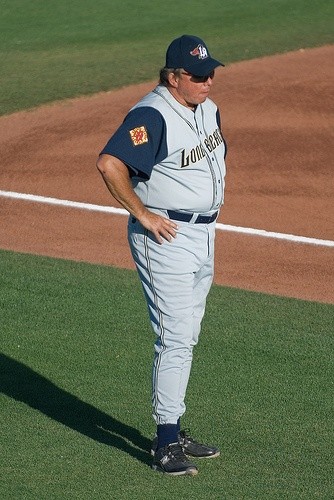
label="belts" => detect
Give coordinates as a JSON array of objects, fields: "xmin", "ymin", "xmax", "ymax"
[{"xmin": 167, "ymin": 208, "xmax": 218, "ymax": 224}]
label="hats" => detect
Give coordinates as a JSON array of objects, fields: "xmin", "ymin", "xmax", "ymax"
[{"xmin": 165, "ymin": 34, "xmax": 226, "ymax": 77}]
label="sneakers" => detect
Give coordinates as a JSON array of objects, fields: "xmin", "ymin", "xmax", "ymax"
[
  {"xmin": 151, "ymin": 437, "xmax": 198, "ymax": 476},
  {"xmin": 177, "ymin": 428, "xmax": 221, "ymax": 459}
]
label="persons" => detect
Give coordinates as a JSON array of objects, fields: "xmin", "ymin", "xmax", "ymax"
[{"xmin": 97, "ymin": 35, "xmax": 228, "ymax": 477}]
